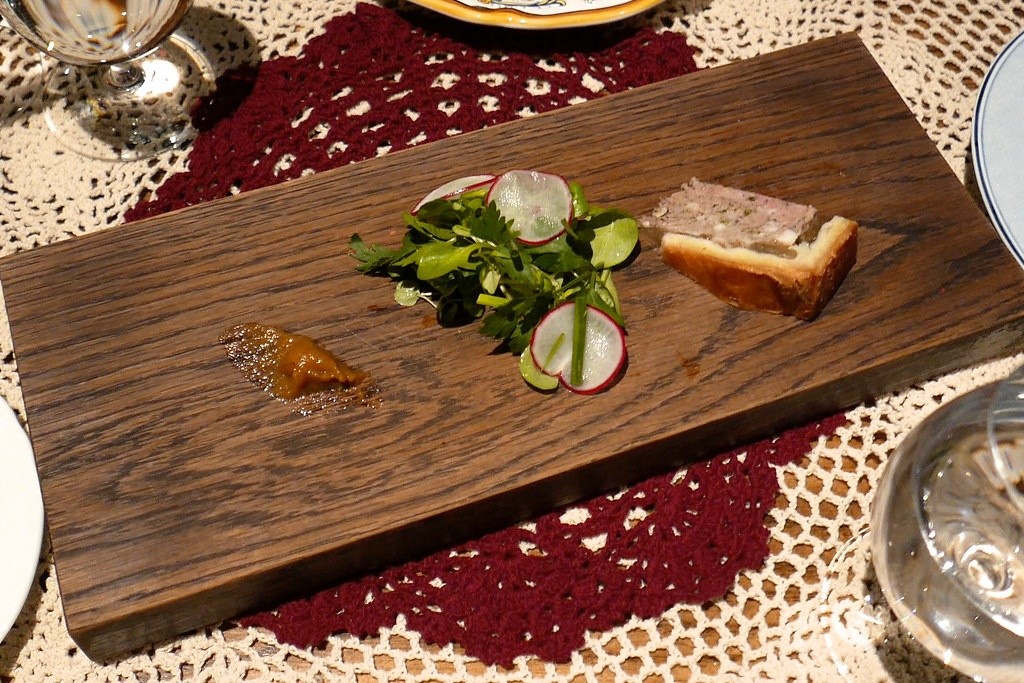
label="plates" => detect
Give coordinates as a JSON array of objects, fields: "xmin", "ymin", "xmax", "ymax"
[
  {"xmin": 405, "ymin": 0, "xmax": 666, "ymax": 30},
  {"xmin": 0, "ymin": 398, "xmax": 45, "ymax": 647},
  {"xmin": 973, "ymin": 31, "xmax": 1023, "ymax": 269}
]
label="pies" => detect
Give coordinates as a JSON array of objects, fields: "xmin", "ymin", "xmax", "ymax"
[{"xmin": 640, "ymin": 177, "xmax": 859, "ymax": 321}]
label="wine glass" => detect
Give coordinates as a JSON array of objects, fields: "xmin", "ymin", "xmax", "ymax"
[
  {"xmin": 819, "ymin": 366, "xmax": 1023, "ymax": 683},
  {"xmin": 0, "ymin": 0, "xmax": 215, "ymax": 162}
]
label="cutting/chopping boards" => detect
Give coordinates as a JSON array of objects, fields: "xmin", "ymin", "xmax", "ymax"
[{"xmin": 2, "ymin": 32, "xmax": 1024, "ymax": 668}]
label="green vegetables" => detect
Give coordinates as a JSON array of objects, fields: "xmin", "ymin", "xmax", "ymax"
[{"xmin": 347, "ymin": 179, "xmax": 639, "ymax": 391}]
